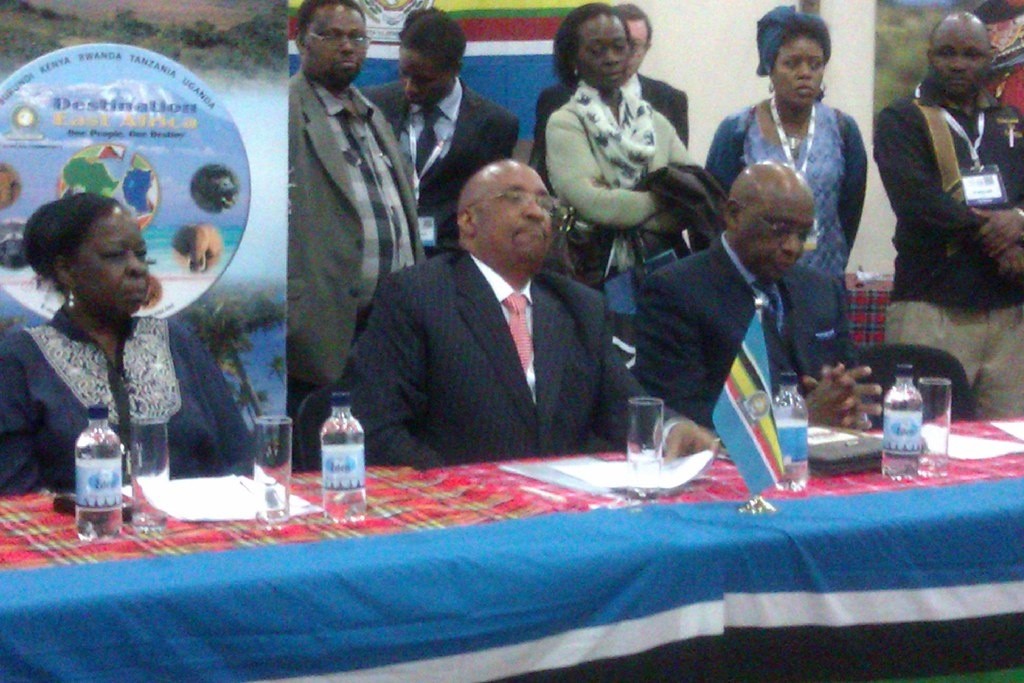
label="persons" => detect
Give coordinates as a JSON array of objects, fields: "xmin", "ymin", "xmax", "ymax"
[
  {"xmin": 344, "ymin": 159, "xmax": 722, "ymax": 466},
  {"xmin": 637, "ymin": 160, "xmax": 895, "ymax": 431},
  {"xmin": 362, "ymin": 8, "xmax": 520, "ymax": 258},
  {"xmin": 530, "ymin": 5, "xmax": 690, "ymax": 284},
  {"xmin": 875, "ymin": 12, "xmax": 1024, "ymax": 419},
  {"xmin": 0, "ymin": 193, "xmax": 254, "ymax": 497},
  {"xmin": 704, "ymin": 8, "xmax": 868, "ymax": 382},
  {"xmin": 288, "ymin": 1, "xmax": 422, "ymax": 419}
]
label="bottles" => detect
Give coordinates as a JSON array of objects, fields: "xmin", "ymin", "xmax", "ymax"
[
  {"xmin": 75, "ymin": 403, "xmax": 120, "ymax": 542},
  {"xmin": 882, "ymin": 364, "xmax": 925, "ymax": 485},
  {"xmin": 320, "ymin": 391, "xmax": 366, "ymax": 524},
  {"xmin": 772, "ymin": 372, "xmax": 809, "ymax": 490}
]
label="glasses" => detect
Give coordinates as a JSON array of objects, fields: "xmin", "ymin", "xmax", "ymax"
[
  {"xmin": 464, "ymin": 189, "xmax": 556, "ymax": 215},
  {"xmin": 307, "ymin": 32, "xmax": 371, "ymax": 49},
  {"xmin": 741, "ymin": 202, "xmax": 821, "ymax": 243}
]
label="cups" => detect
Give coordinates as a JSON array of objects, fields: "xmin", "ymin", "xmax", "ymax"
[
  {"xmin": 918, "ymin": 376, "xmax": 952, "ymax": 477},
  {"xmin": 626, "ymin": 398, "xmax": 664, "ymax": 484},
  {"xmin": 130, "ymin": 415, "xmax": 170, "ymax": 537},
  {"xmin": 255, "ymin": 416, "xmax": 293, "ymax": 526}
]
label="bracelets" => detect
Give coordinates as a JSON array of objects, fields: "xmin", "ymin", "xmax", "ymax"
[{"xmin": 1015, "ymin": 207, "xmax": 1024, "ymax": 216}]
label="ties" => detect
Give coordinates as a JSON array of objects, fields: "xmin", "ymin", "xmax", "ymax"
[
  {"xmin": 416, "ymin": 104, "xmax": 444, "ymax": 176},
  {"xmin": 503, "ymin": 293, "xmax": 533, "ymax": 379},
  {"xmin": 754, "ymin": 282, "xmax": 798, "ymax": 398}
]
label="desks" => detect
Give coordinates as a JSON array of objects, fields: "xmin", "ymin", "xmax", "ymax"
[
  {"xmin": 0, "ymin": 416, "xmax": 1023, "ymax": 683},
  {"xmin": 833, "ymin": 271, "xmax": 907, "ymax": 350}
]
